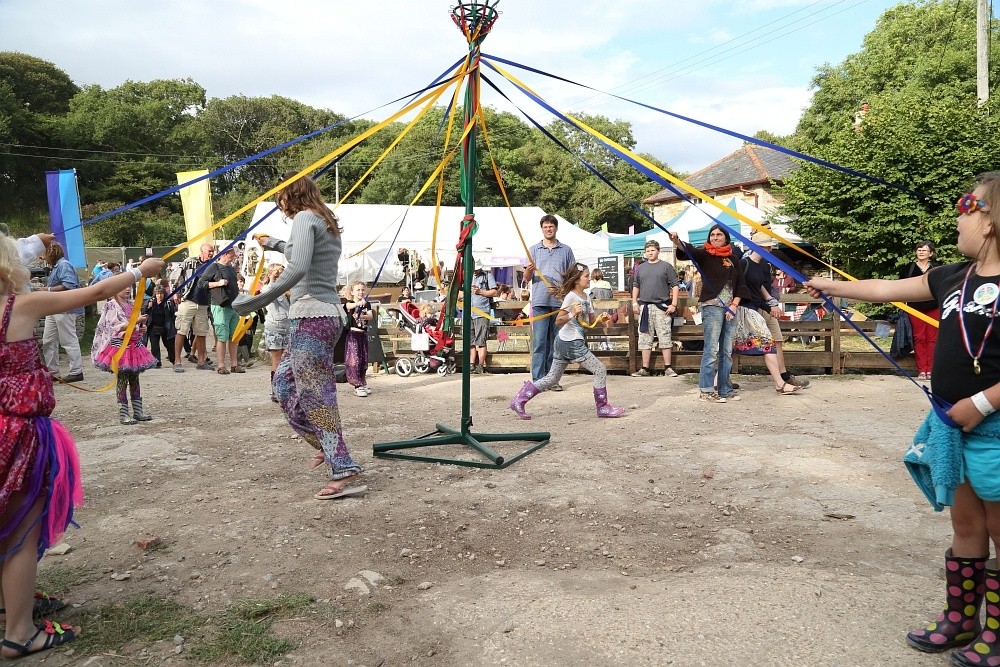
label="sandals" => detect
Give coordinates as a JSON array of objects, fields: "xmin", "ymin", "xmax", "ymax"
[
  {"xmin": 776, "ymin": 382, "xmax": 803, "ymax": 394},
  {"xmin": 0, "ymin": 619, "xmax": 82, "ymax": 661},
  {"xmin": 918, "ymin": 371, "xmax": 931, "ymax": 380},
  {"xmin": 0, "ymin": 588, "xmax": 71, "ymax": 631}
]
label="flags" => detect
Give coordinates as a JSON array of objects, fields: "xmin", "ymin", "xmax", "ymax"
[
  {"xmin": 45, "ymin": 169, "xmax": 87, "ymax": 269},
  {"xmin": 176, "ymin": 170, "xmax": 213, "ymax": 258}
]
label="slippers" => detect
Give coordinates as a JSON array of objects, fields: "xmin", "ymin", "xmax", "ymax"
[
  {"xmin": 307, "ymin": 449, "xmax": 328, "ymax": 472},
  {"xmin": 316, "ymin": 485, "xmax": 367, "ymax": 499}
]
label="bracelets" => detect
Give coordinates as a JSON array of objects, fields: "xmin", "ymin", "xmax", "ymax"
[
  {"xmin": 731, "ymin": 303, "xmax": 738, "ymax": 307},
  {"xmin": 671, "ymin": 304, "xmax": 677, "ymax": 307},
  {"xmin": 971, "ymin": 391, "xmax": 996, "ymax": 417},
  {"xmin": 48, "ymin": 287, "xmax": 51, "ymax": 292},
  {"xmin": 633, "ymin": 302, "xmax": 638, "ymax": 306}
]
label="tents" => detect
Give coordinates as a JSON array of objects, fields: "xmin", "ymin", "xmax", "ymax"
[
  {"xmin": 609, "ymin": 195, "xmax": 811, "ymax": 255},
  {"xmin": 242, "ymin": 199, "xmax": 613, "ymax": 294}
]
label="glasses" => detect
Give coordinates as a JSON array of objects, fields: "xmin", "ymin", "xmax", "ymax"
[{"xmin": 956, "ymin": 193, "xmax": 991, "ymax": 216}]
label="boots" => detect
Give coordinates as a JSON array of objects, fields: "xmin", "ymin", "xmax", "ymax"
[
  {"xmin": 906, "ymin": 546, "xmax": 990, "ymax": 653},
  {"xmin": 949, "ymin": 558, "xmax": 1000, "ymax": 667},
  {"xmin": 118, "ymin": 400, "xmax": 137, "ymax": 425},
  {"xmin": 131, "ymin": 397, "xmax": 152, "ymax": 421},
  {"xmin": 594, "ymin": 386, "xmax": 625, "ymax": 417},
  {"xmin": 509, "ymin": 380, "xmax": 540, "ymax": 419}
]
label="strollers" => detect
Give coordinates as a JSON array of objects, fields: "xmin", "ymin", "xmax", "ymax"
[{"xmin": 386, "ymin": 302, "xmax": 457, "ymax": 377}]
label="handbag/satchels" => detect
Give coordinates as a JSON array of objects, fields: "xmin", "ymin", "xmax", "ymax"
[
  {"xmin": 411, "ymin": 323, "xmax": 430, "ymax": 351},
  {"xmin": 889, "ymin": 308, "xmax": 914, "ymax": 359}
]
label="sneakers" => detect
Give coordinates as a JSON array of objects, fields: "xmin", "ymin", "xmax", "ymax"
[
  {"xmin": 470, "ymin": 364, "xmax": 483, "ymax": 374},
  {"xmin": 786, "ymin": 375, "xmax": 809, "ymax": 386},
  {"xmin": 473, "ymin": 257, "xmax": 484, "ymax": 271},
  {"xmin": 354, "ymin": 386, "xmax": 367, "ymax": 396},
  {"xmin": 631, "ymin": 368, "xmax": 650, "ymax": 377},
  {"xmin": 719, "ymin": 393, "xmax": 741, "ymax": 401},
  {"xmin": 699, "ymin": 391, "xmax": 726, "ymax": 402},
  {"xmin": 665, "ymin": 368, "xmax": 677, "ymax": 376},
  {"xmin": 363, "ymin": 385, "xmax": 372, "ymax": 394}
]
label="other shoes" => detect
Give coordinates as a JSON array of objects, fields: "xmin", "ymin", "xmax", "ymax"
[
  {"xmin": 547, "ymin": 383, "xmax": 563, "ymax": 391},
  {"xmin": 48, "ymin": 371, "xmax": 61, "ymax": 382},
  {"xmin": 714, "ymin": 381, "xmax": 740, "ymax": 390},
  {"xmin": 59, "ymin": 372, "xmax": 84, "ymax": 384}
]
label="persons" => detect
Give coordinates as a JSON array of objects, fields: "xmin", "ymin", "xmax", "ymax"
[
  {"xmin": 670, "ymin": 223, "xmax": 810, "ymax": 403},
  {"xmin": 509, "ymin": 262, "xmax": 625, "ymax": 420},
  {"xmin": 397, "ymin": 257, "xmax": 531, "ymax": 374},
  {"xmin": 0, "ymin": 233, "xmax": 166, "ymax": 659},
  {"xmin": 631, "ymin": 238, "xmax": 679, "ymax": 376},
  {"xmin": 11, "ymin": 233, "xmax": 57, "ymax": 265},
  {"xmin": 522, "ymin": 215, "xmax": 575, "ymax": 391},
  {"xmin": 36, "ymin": 241, "xmax": 291, "ymax": 425},
  {"xmin": 804, "ymin": 172, "xmax": 1000, "ymax": 667},
  {"xmin": 343, "ymin": 280, "xmax": 373, "ymax": 396},
  {"xmin": 231, "ymin": 170, "xmax": 367, "ymax": 500},
  {"xmin": 588, "ymin": 268, "xmax": 614, "ymax": 299},
  {"xmin": 899, "ymin": 241, "xmax": 942, "ymax": 380}
]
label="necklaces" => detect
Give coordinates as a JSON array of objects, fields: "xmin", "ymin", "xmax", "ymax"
[{"xmin": 959, "ymin": 262, "xmax": 1000, "ymax": 374}]
